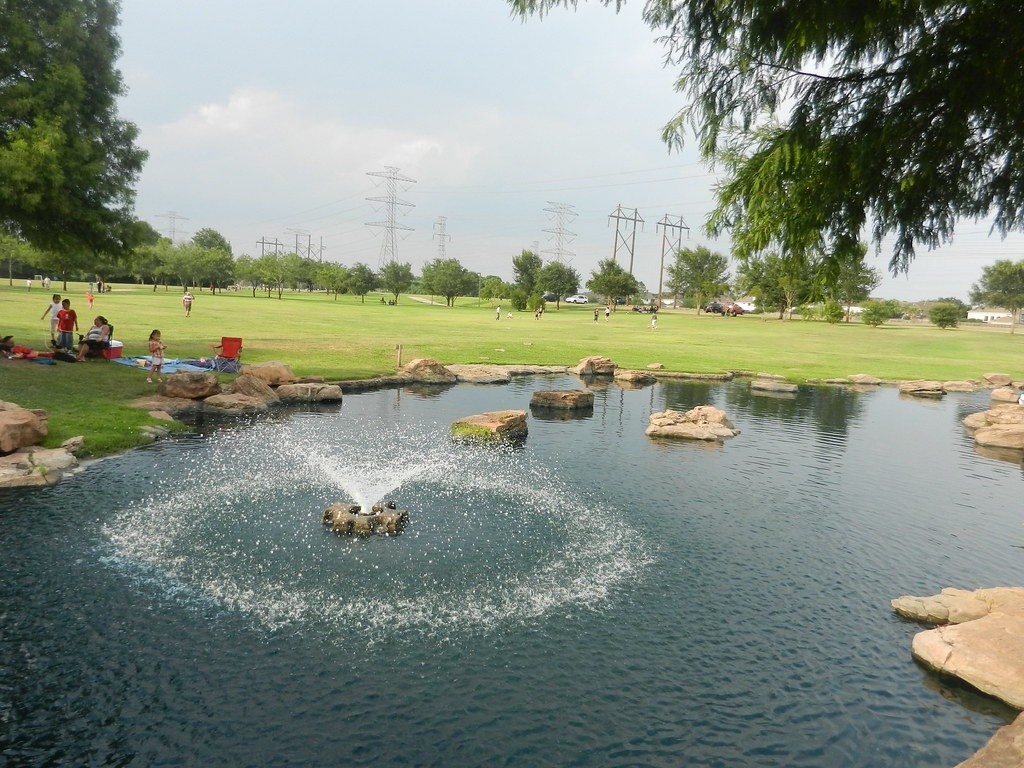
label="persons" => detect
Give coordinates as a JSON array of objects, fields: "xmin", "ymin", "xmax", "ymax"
[
  {"xmin": 146, "ymin": 329, "xmax": 167, "ymax": 384},
  {"xmin": 592, "ymin": 308, "xmax": 599, "ymax": 323},
  {"xmin": 56, "ymin": 299, "xmax": 79, "ymax": 354},
  {"xmin": 533, "ymin": 304, "xmax": 545, "ymax": 321},
  {"xmin": 494, "ymin": 305, "xmax": 500, "ymax": 320},
  {"xmin": 76, "ymin": 315, "xmax": 110, "ymax": 362},
  {"xmin": 42, "ymin": 293, "xmax": 64, "ymax": 343},
  {"xmin": 42, "ymin": 275, "xmax": 51, "ymax": 290},
  {"xmin": 210, "ymin": 283, "xmax": 213, "ymax": 290},
  {"xmin": 89, "ymin": 279, "xmax": 112, "ymax": 295},
  {"xmin": 507, "ymin": 311, "xmax": 513, "ymax": 319},
  {"xmin": 605, "ymin": 307, "xmax": 610, "ymax": 322},
  {"xmin": 86, "ymin": 291, "xmax": 94, "ymax": 312},
  {"xmin": 651, "ymin": 315, "xmax": 657, "ymax": 326},
  {"xmin": 26, "ymin": 277, "xmax": 32, "ymax": 292},
  {"xmin": 630, "ymin": 304, "xmax": 659, "ymax": 314},
  {"xmin": 182, "ymin": 291, "xmax": 196, "ymax": 317}
]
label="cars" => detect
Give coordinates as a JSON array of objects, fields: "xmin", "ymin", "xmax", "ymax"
[
  {"xmin": 543, "ymin": 294, "xmax": 560, "ymax": 302},
  {"xmin": 722, "ymin": 303, "xmax": 745, "ymax": 315},
  {"xmin": 706, "ymin": 302, "xmax": 722, "ymax": 313},
  {"xmin": 566, "ymin": 295, "xmax": 588, "ymax": 304}
]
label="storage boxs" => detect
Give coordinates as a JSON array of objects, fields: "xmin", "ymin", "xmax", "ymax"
[{"xmin": 103, "ymin": 340, "xmax": 124, "ymax": 359}]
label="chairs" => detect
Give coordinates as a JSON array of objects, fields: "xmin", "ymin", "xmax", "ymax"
[
  {"xmin": 214, "ymin": 337, "xmax": 243, "ymax": 374},
  {"xmin": 89, "ymin": 324, "xmax": 114, "ymax": 362}
]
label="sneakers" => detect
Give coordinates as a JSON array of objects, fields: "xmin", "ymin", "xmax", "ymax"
[
  {"xmin": 158, "ymin": 378, "xmax": 163, "ymax": 383},
  {"xmin": 146, "ymin": 377, "xmax": 153, "ymax": 383}
]
